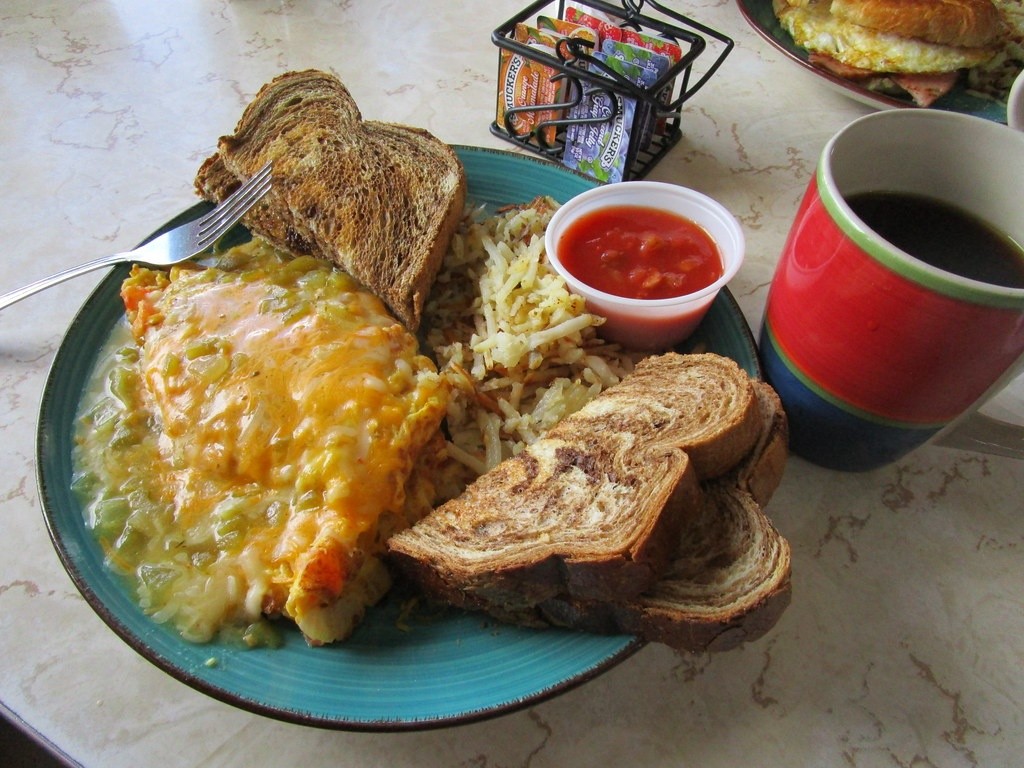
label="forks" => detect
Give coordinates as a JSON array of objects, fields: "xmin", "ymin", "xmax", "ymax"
[{"xmin": 0, "ymin": 160, "xmax": 273, "ymax": 306}]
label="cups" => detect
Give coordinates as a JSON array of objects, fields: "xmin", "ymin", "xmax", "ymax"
[{"xmin": 760, "ymin": 111, "xmax": 1024, "ymax": 472}]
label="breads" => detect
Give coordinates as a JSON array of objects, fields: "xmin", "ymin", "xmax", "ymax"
[
  {"xmin": 391, "ymin": 352, "xmax": 764, "ymax": 603},
  {"xmin": 484, "ymin": 382, "xmax": 795, "ymax": 646},
  {"xmin": 217, "ymin": 64, "xmax": 464, "ymax": 316},
  {"xmin": 192, "ymin": 156, "xmax": 317, "ymax": 261}
]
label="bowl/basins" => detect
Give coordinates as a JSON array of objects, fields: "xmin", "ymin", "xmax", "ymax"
[{"xmin": 545, "ymin": 181, "xmax": 745, "ymax": 351}]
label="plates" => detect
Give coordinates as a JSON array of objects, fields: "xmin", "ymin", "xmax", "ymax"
[
  {"xmin": 36, "ymin": 145, "xmax": 762, "ymax": 733},
  {"xmin": 735, "ymin": 0, "xmax": 1008, "ymax": 126}
]
